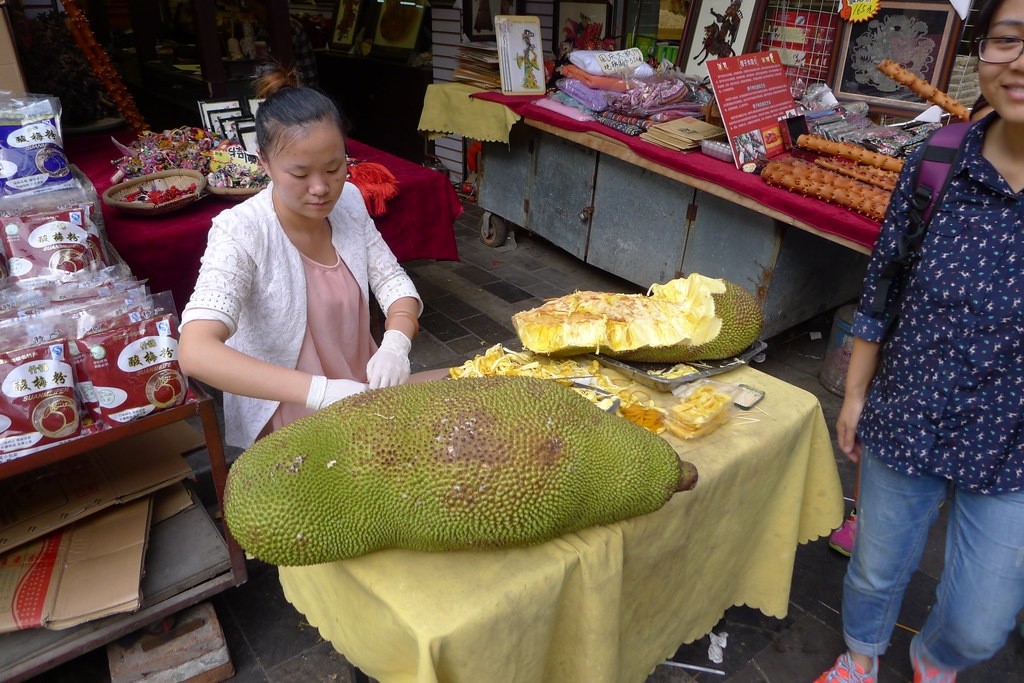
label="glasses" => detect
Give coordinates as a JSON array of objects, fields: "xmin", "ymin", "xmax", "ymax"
[{"xmin": 976, "ymin": 29, "xmax": 1024, "ymax": 66}]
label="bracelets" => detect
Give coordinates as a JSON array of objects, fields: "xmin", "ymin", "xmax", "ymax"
[{"xmin": 384, "ymin": 310, "xmax": 419, "ymax": 333}]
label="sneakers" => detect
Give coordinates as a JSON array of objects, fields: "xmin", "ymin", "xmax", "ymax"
[
  {"xmin": 827, "ymin": 507, "xmax": 860, "ymax": 558},
  {"xmin": 908, "ymin": 633, "xmax": 958, "ymax": 683},
  {"xmin": 811, "ymin": 650, "xmax": 880, "ymax": 683}
]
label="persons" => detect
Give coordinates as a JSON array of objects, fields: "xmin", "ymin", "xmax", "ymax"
[
  {"xmin": 809, "ymin": 0, "xmax": 1024, "ymax": 683},
  {"xmin": 830, "ymin": 97, "xmax": 993, "ymax": 556},
  {"xmin": 176, "ymin": 69, "xmax": 423, "ymax": 449}
]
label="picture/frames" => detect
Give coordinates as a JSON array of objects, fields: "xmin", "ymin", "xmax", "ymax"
[
  {"xmin": 832, "ymin": 1, "xmax": 964, "ymax": 111},
  {"xmin": 495, "ymin": 15, "xmax": 546, "ymax": 95},
  {"xmin": 674, "ymin": 0, "xmax": 768, "ymax": 77}
]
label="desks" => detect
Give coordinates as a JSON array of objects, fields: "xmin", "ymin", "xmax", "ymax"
[
  {"xmin": 63, "ymin": 120, "xmax": 462, "ymax": 328},
  {"xmin": 0, "ymin": 378, "xmax": 251, "ymax": 683},
  {"xmin": 277, "ymin": 361, "xmax": 852, "ymax": 682}
]
